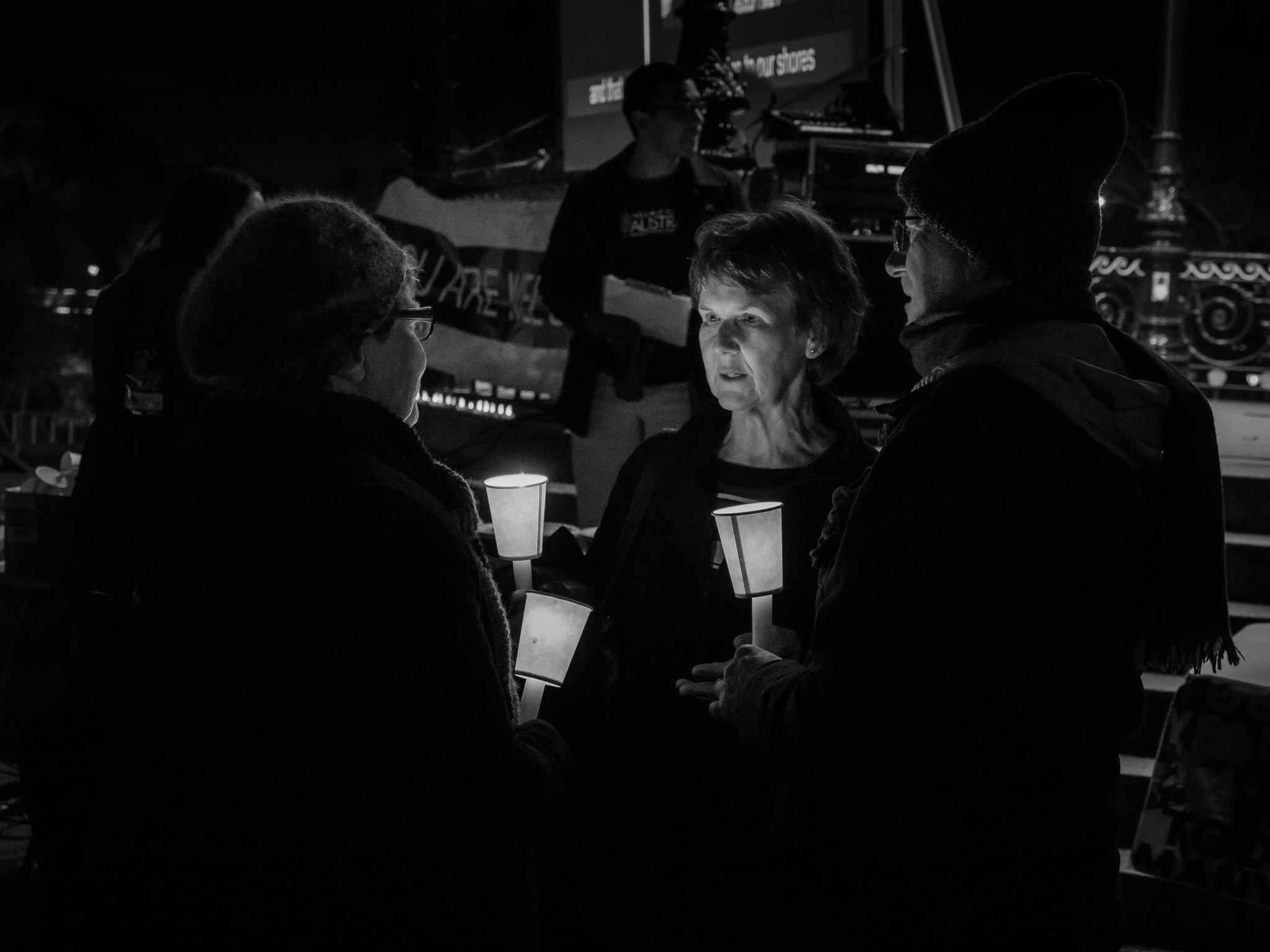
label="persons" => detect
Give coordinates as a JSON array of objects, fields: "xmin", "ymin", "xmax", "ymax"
[
  {"xmin": 17, "ymin": 197, "xmax": 574, "ymax": 952},
  {"xmin": 90, "ymin": 169, "xmax": 268, "ymax": 423},
  {"xmin": 540, "ymin": 61, "xmax": 755, "ymax": 531},
  {"xmin": 561, "ymin": 201, "xmax": 874, "ymax": 952},
  {"xmin": 674, "ymin": 74, "xmax": 1227, "ymax": 952}
]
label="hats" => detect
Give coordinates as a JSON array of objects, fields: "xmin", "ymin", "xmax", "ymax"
[
  {"xmin": 182, "ymin": 199, "xmax": 411, "ymax": 381},
  {"xmin": 897, "ymin": 73, "xmax": 1126, "ymax": 283}
]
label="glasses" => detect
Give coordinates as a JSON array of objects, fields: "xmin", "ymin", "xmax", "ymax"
[
  {"xmin": 384, "ymin": 303, "xmax": 440, "ymax": 342},
  {"xmin": 890, "ymin": 213, "xmax": 928, "ymax": 253}
]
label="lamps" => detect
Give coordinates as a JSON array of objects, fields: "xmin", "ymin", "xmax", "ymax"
[
  {"xmin": 514, "ymin": 590, "xmax": 593, "ymax": 728},
  {"xmin": 711, "ymin": 500, "xmax": 785, "ymax": 654},
  {"xmin": 484, "ymin": 472, "xmax": 548, "ymax": 595}
]
